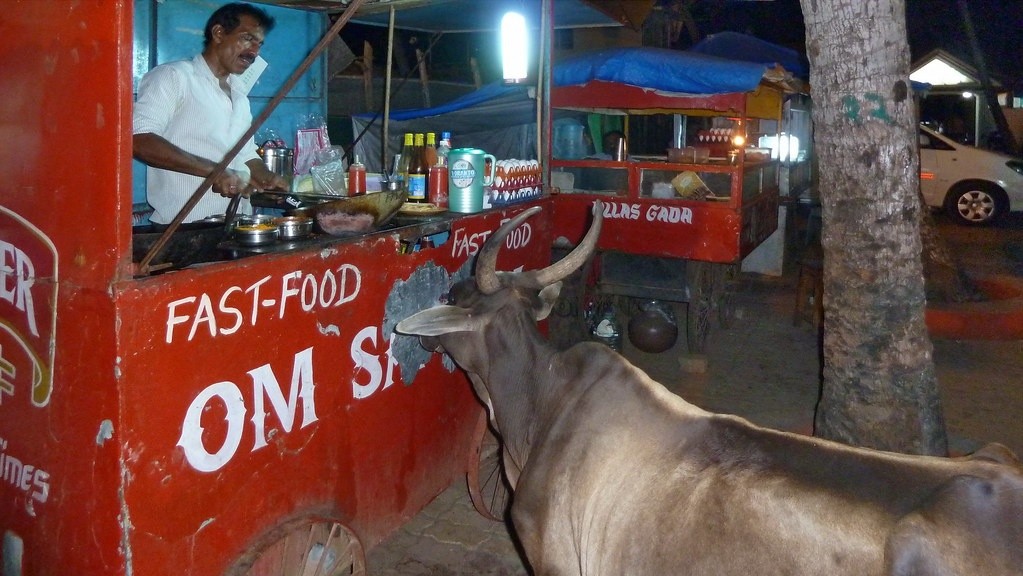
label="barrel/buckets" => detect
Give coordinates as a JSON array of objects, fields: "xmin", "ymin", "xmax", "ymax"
[{"xmin": 263, "ymin": 148, "xmax": 294, "ymax": 189}]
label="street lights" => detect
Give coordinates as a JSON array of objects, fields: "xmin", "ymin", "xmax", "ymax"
[{"xmin": 963, "ymin": 90, "xmax": 979, "ymax": 146}]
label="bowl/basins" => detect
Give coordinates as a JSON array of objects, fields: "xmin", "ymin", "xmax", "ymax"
[
  {"xmin": 270, "ymin": 216, "xmax": 314, "ymax": 240},
  {"xmin": 235, "ymin": 226, "xmax": 279, "ymax": 245}
]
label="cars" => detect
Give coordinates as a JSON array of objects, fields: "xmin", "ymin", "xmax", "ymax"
[{"xmin": 920, "ymin": 123, "xmax": 1023, "ymax": 224}]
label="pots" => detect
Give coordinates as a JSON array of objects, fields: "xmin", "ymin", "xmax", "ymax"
[{"xmin": 273, "ymin": 187, "xmax": 405, "ymax": 237}]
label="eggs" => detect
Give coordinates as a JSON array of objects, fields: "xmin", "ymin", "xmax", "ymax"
[
  {"xmin": 483, "ymin": 158, "xmax": 539, "ymax": 201},
  {"xmin": 699, "ymin": 128, "xmax": 733, "ymax": 142}
]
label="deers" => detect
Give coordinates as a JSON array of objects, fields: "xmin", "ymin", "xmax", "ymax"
[{"xmin": 395, "ymin": 197, "xmax": 1023, "ymax": 575}]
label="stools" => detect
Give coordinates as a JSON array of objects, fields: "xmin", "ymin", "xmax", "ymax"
[{"xmin": 792, "ymin": 252, "xmax": 823, "ymax": 337}]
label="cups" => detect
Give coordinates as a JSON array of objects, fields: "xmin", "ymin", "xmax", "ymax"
[
  {"xmin": 381, "ymin": 180, "xmax": 405, "ymax": 192},
  {"xmin": 613, "ymin": 138, "xmax": 627, "ymax": 162}
]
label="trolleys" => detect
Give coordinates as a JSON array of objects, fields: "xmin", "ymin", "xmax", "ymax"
[
  {"xmin": 551, "ymin": 33, "xmax": 817, "ymax": 353},
  {"xmin": 1, "ymin": 1, "xmax": 630, "ymax": 576}
]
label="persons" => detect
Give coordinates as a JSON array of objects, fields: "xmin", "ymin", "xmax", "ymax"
[
  {"xmin": 578, "ymin": 131, "xmax": 631, "ymax": 189},
  {"xmin": 133, "ymin": 3, "xmax": 291, "ymax": 266}
]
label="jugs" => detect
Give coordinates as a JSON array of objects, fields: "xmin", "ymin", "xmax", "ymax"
[
  {"xmin": 554, "ymin": 125, "xmax": 586, "ymax": 160},
  {"xmin": 446, "ymin": 148, "xmax": 496, "ymax": 212}
]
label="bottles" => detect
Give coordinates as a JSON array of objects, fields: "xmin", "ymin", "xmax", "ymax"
[
  {"xmin": 397, "ymin": 133, "xmax": 414, "ymax": 201},
  {"xmin": 348, "ymin": 155, "xmax": 366, "ymax": 197},
  {"xmin": 585, "ymin": 310, "xmax": 597, "ymax": 328},
  {"xmin": 430, "ymin": 157, "xmax": 447, "ymax": 208},
  {"xmin": 442, "ymin": 132, "xmax": 451, "ymax": 148},
  {"xmin": 425, "ymin": 133, "xmax": 437, "ymax": 166},
  {"xmin": 437, "ymin": 140, "xmax": 450, "ymax": 156},
  {"xmin": 593, "ymin": 306, "xmax": 623, "ymax": 354},
  {"xmin": 408, "ymin": 134, "xmax": 428, "ymax": 203},
  {"xmin": 391, "ymin": 153, "xmax": 404, "ymax": 181}
]
